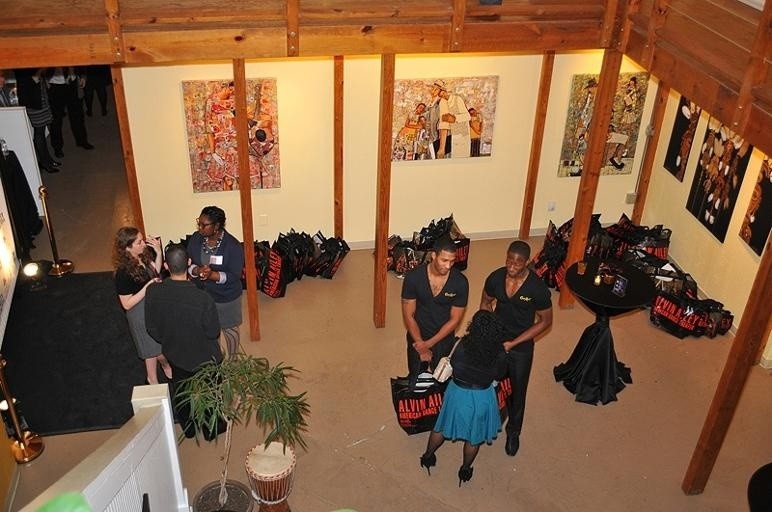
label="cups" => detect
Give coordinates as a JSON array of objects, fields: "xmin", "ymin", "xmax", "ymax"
[{"xmin": 577, "ymin": 259, "xmax": 586, "ymax": 275}]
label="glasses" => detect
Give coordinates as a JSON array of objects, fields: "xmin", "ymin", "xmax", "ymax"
[{"xmin": 196, "ymin": 216, "xmax": 217, "ymax": 229}]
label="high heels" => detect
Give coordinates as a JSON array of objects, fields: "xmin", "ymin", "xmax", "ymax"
[
  {"xmin": 458, "ymin": 464, "xmax": 475, "ymax": 486},
  {"xmin": 419, "ymin": 452, "xmax": 436, "ymax": 475},
  {"xmin": 38, "ymin": 156, "xmax": 62, "ymax": 176}
]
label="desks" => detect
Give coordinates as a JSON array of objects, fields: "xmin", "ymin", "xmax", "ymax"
[{"xmin": 553, "ymin": 258, "xmax": 658, "ymax": 405}]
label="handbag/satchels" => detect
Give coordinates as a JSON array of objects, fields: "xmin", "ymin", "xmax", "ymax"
[
  {"xmin": 390, "ymin": 359, "xmax": 445, "ymax": 436},
  {"xmin": 373, "ymin": 214, "xmax": 471, "ymax": 278},
  {"xmin": 433, "ymin": 355, "xmax": 455, "ymax": 384},
  {"xmin": 239, "ymin": 228, "xmax": 350, "ymax": 299},
  {"xmin": 493, "ymin": 377, "xmax": 515, "ymax": 428}
]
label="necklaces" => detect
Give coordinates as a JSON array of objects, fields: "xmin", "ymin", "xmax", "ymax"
[{"xmin": 202, "ymin": 237, "xmax": 222, "ymax": 254}]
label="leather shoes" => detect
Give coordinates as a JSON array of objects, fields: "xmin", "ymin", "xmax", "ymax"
[
  {"xmin": 202, "ymin": 416, "xmax": 232, "ymax": 440},
  {"xmin": 527, "ymin": 212, "xmax": 734, "ymax": 340},
  {"xmin": 54, "ymin": 145, "xmax": 66, "ymax": 157},
  {"xmin": 178, "ymin": 414, "xmax": 197, "ymax": 438},
  {"xmin": 75, "ymin": 141, "xmax": 96, "ymax": 151},
  {"xmin": 145, "ymin": 363, "xmax": 173, "ymax": 385},
  {"xmin": 506, "ymin": 431, "xmax": 521, "ymax": 457}
]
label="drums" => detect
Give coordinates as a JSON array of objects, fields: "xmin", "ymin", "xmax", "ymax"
[{"xmin": 245, "ymin": 441, "xmax": 296, "ymax": 511}]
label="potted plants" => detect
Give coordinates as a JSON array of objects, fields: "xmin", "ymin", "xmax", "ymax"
[{"xmin": 175, "ymin": 347, "xmax": 310, "ymax": 512}]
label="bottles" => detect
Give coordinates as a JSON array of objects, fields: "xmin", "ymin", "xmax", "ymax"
[{"xmin": 594, "ymin": 260, "xmax": 617, "ymax": 285}]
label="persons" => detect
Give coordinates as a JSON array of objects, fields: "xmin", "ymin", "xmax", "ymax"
[
  {"xmin": 14, "ymin": 67, "xmax": 108, "ymax": 174},
  {"xmin": 391, "ymin": 78, "xmax": 492, "ymax": 161},
  {"xmin": 184, "ymin": 205, "xmax": 245, "ymax": 361},
  {"xmin": 479, "ymin": 241, "xmax": 553, "ymax": 456},
  {"xmin": 401, "ymin": 238, "xmax": 469, "ymax": 374},
  {"xmin": 144, "ymin": 247, "xmax": 228, "ymax": 442},
  {"xmin": 571, "ymin": 76, "xmax": 640, "ymax": 177},
  {"xmin": 113, "ymin": 226, "xmax": 173, "ymax": 385},
  {"xmin": 419, "ymin": 309, "xmax": 509, "ymax": 488}
]
